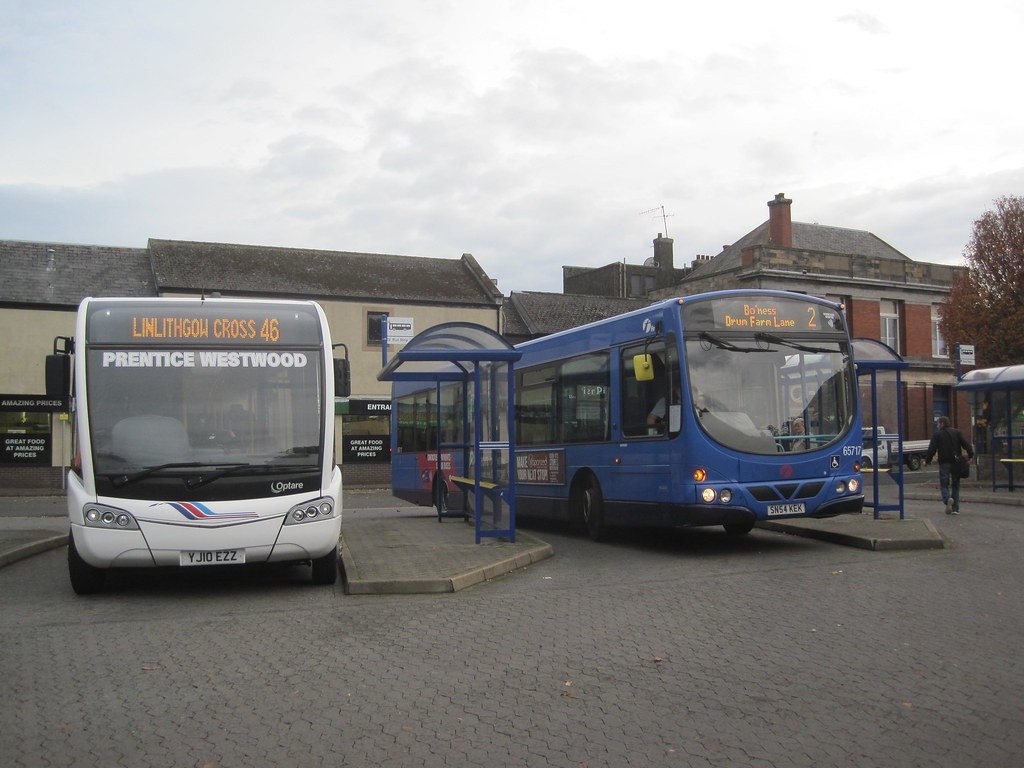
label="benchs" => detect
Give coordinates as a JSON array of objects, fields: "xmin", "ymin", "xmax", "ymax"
[
  {"xmin": 450, "ymin": 475, "xmax": 510, "ymax": 506},
  {"xmin": 860, "ymin": 468, "xmax": 899, "ymax": 486},
  {"xmin": 1000, "ymin": 458, "xmax": 1024, "ymax": 469}
]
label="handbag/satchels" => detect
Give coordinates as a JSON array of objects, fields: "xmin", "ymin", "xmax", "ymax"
[{"xmin": 955, "ymin": 455, "xmax": 969, "ymax": 479}]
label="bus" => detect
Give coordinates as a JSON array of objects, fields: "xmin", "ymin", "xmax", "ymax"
[
  {"xmin": 390, "ymin": 289, "xmax": 865, "ymax": 543},
  {"xmin": 45, "ymin": 291, "xmax": 352, "ymax": 595}
]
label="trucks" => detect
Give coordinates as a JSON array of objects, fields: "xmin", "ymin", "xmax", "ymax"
[{"xmin": 860, "ymin": 426, "xmax": 931, "ymax": 472}]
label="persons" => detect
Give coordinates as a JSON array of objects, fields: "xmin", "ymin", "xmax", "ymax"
[
  {"xmin": 790, "ymin": 417, "xmax": 817, "ymax": 453},
  {"xmin": 646, "ymin": 397, "xmax": 666, "ymax": 429},
  {"xmin": 923, "ymin": 416, "xmax": 975, "ymax": 515}
]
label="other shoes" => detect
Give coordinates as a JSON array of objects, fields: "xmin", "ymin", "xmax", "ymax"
[
  {"xmin": 953, "ymin": 509, "xmax": 960, "ymax": 515},
  {"xmin": 945, "ymin": 498, "xmax": 954, "ymax": 514}
]
